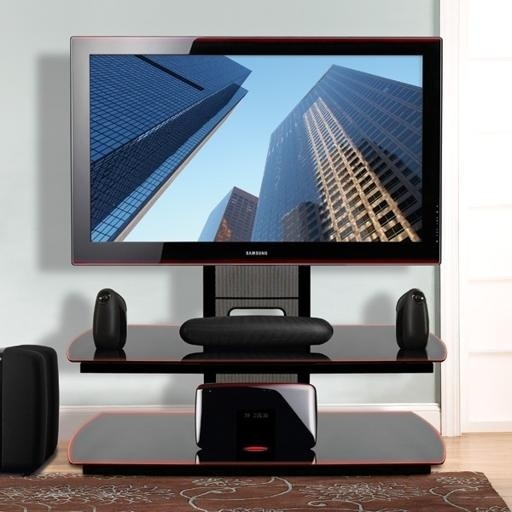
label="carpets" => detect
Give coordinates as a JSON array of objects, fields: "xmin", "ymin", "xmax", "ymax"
[{"xmin": 1, "ymin": 471, "xmax": 510, "ymax": 511}]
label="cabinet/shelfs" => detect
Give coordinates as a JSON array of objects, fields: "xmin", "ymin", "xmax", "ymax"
[{"xmin": 65, "ymin": 323, "xmax": 445, "ymax": 474}]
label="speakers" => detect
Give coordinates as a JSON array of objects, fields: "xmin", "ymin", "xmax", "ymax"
[
  {"xmin": 0, "ymin": 345, "xmax": 61, "ymax": 477},
  {"xmin": 178, "ymin": 317, "xmax": 336, "ymax": 348},
  {"xmin": 395, "ymin": 288, "xmax": 430, "ymax": 352},
  {"xmin": 194, "ymin": 381, "xmax": 318, "ymax": 450},
  {"xmin": 92, "ymin": 287, "xmax": 128, "ymax": 349}
]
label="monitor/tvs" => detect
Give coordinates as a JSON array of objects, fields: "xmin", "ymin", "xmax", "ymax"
[{"xmin": 69, "ymin": 35, "xmax": 441, "ymax": 267}]
